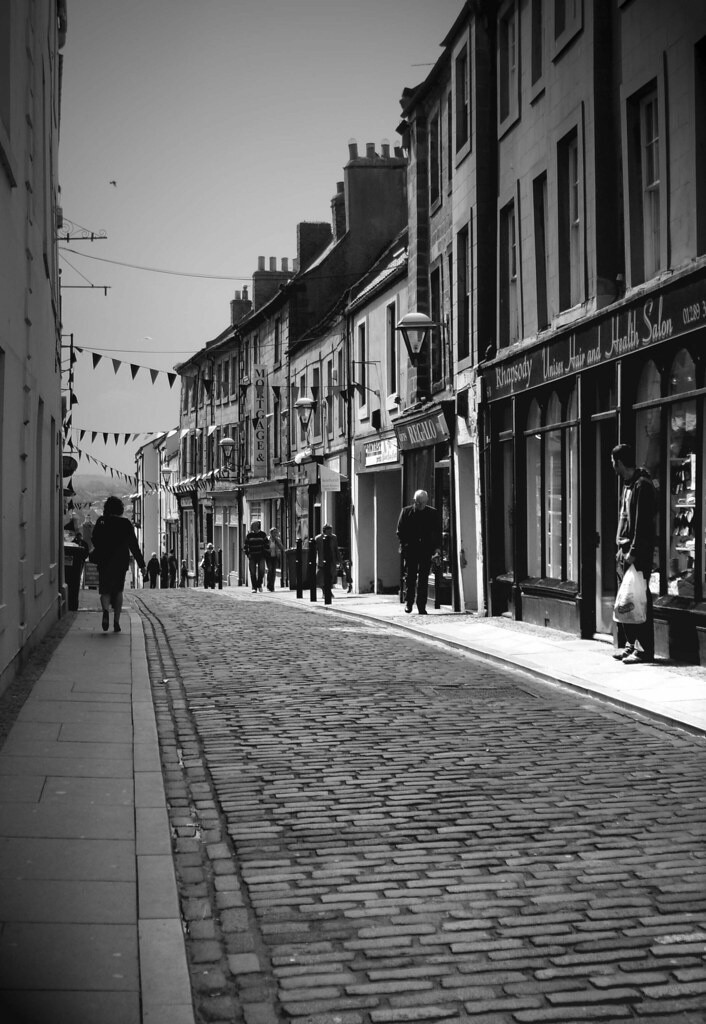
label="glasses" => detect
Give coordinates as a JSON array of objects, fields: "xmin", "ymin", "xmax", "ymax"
[{"xmin": 416, "ymin": 500, "xmax": 427, "ymax": 505}]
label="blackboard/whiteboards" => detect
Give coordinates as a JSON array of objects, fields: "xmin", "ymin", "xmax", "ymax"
[{"xmin": 82, "ymin": 561, "xmax": 100, "ymax": 587}]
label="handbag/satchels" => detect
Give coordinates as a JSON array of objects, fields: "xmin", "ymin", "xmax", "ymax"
[
  {"xmin": 89, "ymin": 516, "xmax": 105, "ymax": 564},
  {"xmin": 612, "ymin": 563, "xmax": 646, "ymax": 624}
]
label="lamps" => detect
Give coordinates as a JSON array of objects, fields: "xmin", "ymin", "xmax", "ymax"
[
  {"xmin": 294, "ymin": 398, "xmax": 318, "ymax": 433},
  {"xmin": 162, "ymin": 467, "xmax": 172, "ymax": 489},
  {"xmin": 219, "ymin": 438, "xmax": 236, "ymax": 459},
  {"xmin": 396, "ymin": 313, "xmax": 439, "ymax": 372}
]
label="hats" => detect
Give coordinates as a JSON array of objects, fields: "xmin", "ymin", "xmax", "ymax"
[{"xmin": 207, "ymin": 545, "xmax": 214, "ymax": 549}]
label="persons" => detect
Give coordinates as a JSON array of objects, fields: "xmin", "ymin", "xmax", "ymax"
[
  {"xmin": 396, "ymin": 490, "xmax": 443, "ymax": 615},
  {"xmin": 89, "ymin": 496, "xmax": 147, "ymax": 632},
  {"xmin": 315, "ymin": 525, "xmax": 343, "ymax": 599},
  {"xmin": 146, "ymin": 550, "xmax": 178, "ymax": 589},
  {"xmin": 72, "ymin": 533, "xmax": 89, "ymax": 581},
  {"xmin": 610, "ymin": 444, "xmax": 658, "ymax": 664},
  {"xmin": 179, "ymin": 561, "xmax": 188, "ymax": 588},
  {"xmin": 199, "ymin": 543, "xmax": 217, "ymax": 590},
  {"xmin": 242, "ymin": 520, "xmax": 284, "ymax": 594}
]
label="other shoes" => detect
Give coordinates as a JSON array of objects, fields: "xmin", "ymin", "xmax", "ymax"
[
  {"xmin": 252, "ymin": 589, "xmax": 256, "ymax": 593},
  {"xmin": 259, "ymin": 587, "xmax": 263, "ymax": 592},
  {"xmin": 114, "ymin": 622, "xmax": 121, "ymax": 632},
  {"xmin": 405, "ymin": 601, "xmax": 413, "ymax": 613},
  {"xmin": 612, "ymin": 646, "xmax": 635, "ymax": 660},
  {"xmin": 622, "ymin": 650, "xmax": 654, "ymax": 664},
  {"xmin": 102, "ymin": 610, "xmax": 109, "ymax": 631},
  {"xmin": 417, "ymin": 604, "xmax": 428, "ymax": 614}
]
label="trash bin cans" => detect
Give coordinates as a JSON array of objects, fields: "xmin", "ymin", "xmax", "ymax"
[
  {"xmin": 64, "ymin": 541, "xmax": 85, "ymax": 611},
  {"xmin": 285, "ymin": 547, "xmax": 310, "ymax": 590}
]
